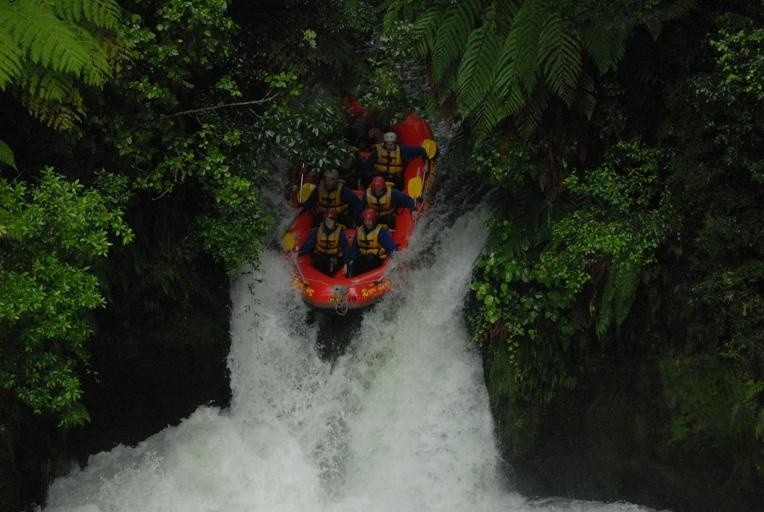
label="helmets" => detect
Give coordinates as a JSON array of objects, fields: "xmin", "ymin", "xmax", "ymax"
[
  {"xmin": 323, "ymin": 208, "xmax": 337, "ymax": 219},
  {"xmin": 323, "ymin": 169, "xmax": 339, "ymax": 181},
  {"xmin": 371, "ymin": 175, "xmax": 385, "ymax": 192},
  {"xmin": 360, "ymin": 208, "xmax": 377, "ymax": 221},
  {"xmin": 383, "ymin": 131, "xmax": 397, "ymax": 142}
]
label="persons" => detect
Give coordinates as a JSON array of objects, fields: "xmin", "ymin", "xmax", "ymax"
[
  {"xmin": 291, "ymin": 210, "xmax": 350, "ymax": 278},
  {"xmin": 366, "ymin": 131, "xmax": 429, "ymax": 191},
  {"xmin": 345, "ymin": 207, "xmax": 399, "ymax": 278},
  {"xmin": 360, "ymin": 176, "xmax": 417, "ymax": 230},
  {"xmin": 296, "ymin": 169, "xmax": 360, "ymax": 229}
]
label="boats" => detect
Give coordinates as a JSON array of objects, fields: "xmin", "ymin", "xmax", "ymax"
[{"xmin": 287, "ymin": 95, "xmax": 439, "ymax": 317}]
